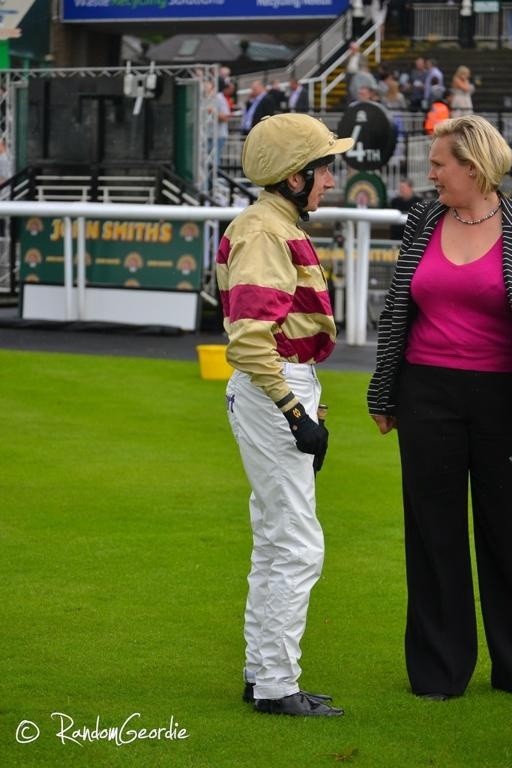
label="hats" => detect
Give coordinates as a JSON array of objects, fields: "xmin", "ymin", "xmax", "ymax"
[{"xmin": 241, "ymin": 111, "xmax": 355, "ymax": 188}]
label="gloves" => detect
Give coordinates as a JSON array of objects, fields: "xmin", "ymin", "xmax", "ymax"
[
  {"xmin": 281, "ymin": 401, "xmax": 325, "ymax": 454},
  {"xmin": 312, "ymin": 404, "xmax": 330, "ymax": 480}
]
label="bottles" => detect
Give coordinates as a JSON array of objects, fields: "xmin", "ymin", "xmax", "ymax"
[
  {"xmin": 322, "ymin": 264, "xmax": 335, "ymax": 281},
  {"xmin": 306, "ymin": 244, "xmax": 399, "ymax": 265}
]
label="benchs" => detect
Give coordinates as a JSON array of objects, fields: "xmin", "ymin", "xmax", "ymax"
[{"xmin": 292, "ymin": 37, "xmax": 511, "ymax": 122}]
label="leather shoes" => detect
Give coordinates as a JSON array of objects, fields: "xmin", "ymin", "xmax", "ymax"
[
  {"xmin": 419, "ymin": 693, "xmax": 452, "ymax": 703},
  {"xmin": 242, "ymin": 681, "xmax": 345, "ymax": 719}
]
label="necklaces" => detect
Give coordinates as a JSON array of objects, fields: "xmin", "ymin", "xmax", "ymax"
[{"xmin": 451, "ymin": 199, "xmax": 501, "ymax": 224}]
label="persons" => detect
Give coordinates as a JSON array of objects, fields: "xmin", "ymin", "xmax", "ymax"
[
  {"xmin": 367, "ymin": 113, "xmax": 510, "ymax": 699},
  {"xmin": 217, "ymin": 111, "xmax": 354, "ymax": 716}
]
